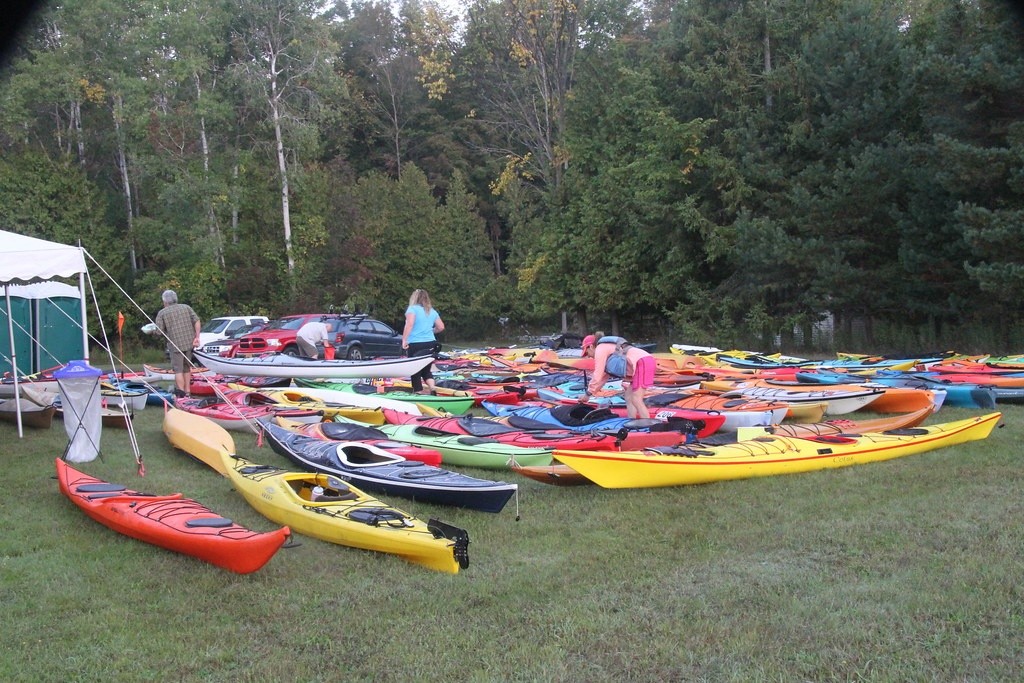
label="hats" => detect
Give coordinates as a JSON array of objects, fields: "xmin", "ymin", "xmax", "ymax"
[{"xmin": 580, "ymin": 335, "xmax": 597, "ymax": 358}]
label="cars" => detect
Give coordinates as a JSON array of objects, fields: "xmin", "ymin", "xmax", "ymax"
[
  {"xmin": 203, "ymin": 321, "xmax": 277, "ymax": 357},
  {"xmin": 165, "ymin": 316, "xmax": 271, "ymax": 357},
  {"xmin": 239, "ymin": 314, "xmax": 338, "ymax": 354}
]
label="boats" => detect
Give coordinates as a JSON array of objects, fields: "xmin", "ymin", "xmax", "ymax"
[
  {"xmin": 253, "ymin": 415, "xmax": 522, "ymax": 517},
  {"xmin": 549, "ymin": 412, "xmax": 1004, "ymax": 490},
  {"xmin": 56, "ymin": 457, "xmax": 292, "ymax": 576},
  {"xmin": 220, "ymin": 445, "xmax": 469, "ymax": 574},
  {"xmin": 0, "ymin": 338, "xmax": 1024, "ymax": 469}
]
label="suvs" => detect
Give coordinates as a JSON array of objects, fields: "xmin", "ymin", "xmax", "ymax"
[{"xmin": 317, "ymin": 314, "xmax": 407, "ymax": 361}]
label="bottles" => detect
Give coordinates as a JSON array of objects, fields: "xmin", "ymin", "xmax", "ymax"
[{"xmin": 312, "ymin": 487, "xmax": 324, "ymax": 502}]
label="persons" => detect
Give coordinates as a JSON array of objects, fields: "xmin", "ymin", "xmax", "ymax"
[
  {"xmin": 296, "ymin": 322, "xmax": 333, "ymax": 359},
  {"xmin": 402, "ymin": 289, "xmax": 444, "ymax": 396},
  {"xmin": 578, "ymin": 334, "xmax": 656, "ymax": 419},
  {"xmin": 152, "ymin": 290, "xmax": 201, "ymax": 398}
]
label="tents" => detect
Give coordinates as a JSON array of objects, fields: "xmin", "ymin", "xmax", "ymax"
[{"xmin": 0, "ymin": 228, "xmax": 89, "ymax": 438}]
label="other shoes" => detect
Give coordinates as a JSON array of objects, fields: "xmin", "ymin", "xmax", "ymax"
[
  {"xmin": 430, "ymin": 388, "xmax": 437, "ymax": 396},
  {"xmin": 414, "ymin": 391, "xmax": 426, "ymax": 395}
]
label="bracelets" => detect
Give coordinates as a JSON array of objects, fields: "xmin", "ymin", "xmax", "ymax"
[{"xmin": 586, "ymin": 391, "xmax": 591, "ymax": 396}]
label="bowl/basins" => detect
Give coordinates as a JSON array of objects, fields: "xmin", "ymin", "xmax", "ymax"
[{"xmin": 141, "ymin": 326, "xmax": 157, "ymax": 334}]
